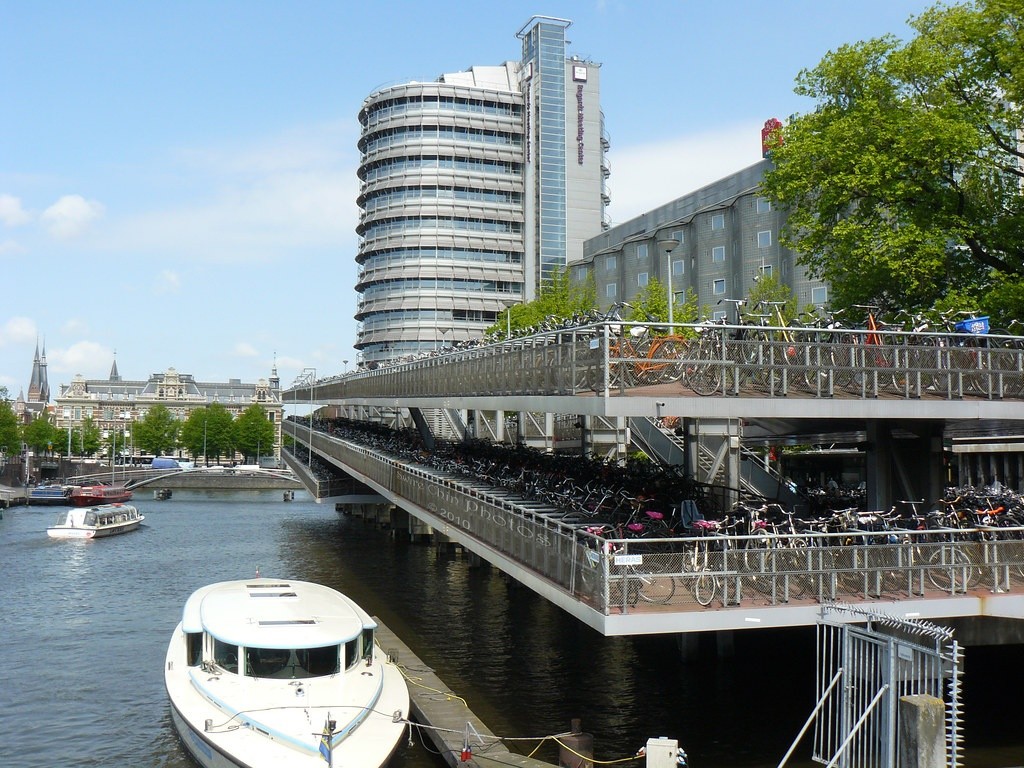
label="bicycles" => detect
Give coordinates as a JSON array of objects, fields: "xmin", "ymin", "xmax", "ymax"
[
  {"xmin": 316, "ymin": 298, "xmax": 1024, "ymax": 397},
  {"xmin": 287, "ymin": 415, "xmax": 1024, "ymax": 606}
]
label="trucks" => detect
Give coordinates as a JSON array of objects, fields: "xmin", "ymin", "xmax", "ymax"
[{"xmin": 232, "ymin": 465, "xmax": 260, "ymax": 476}]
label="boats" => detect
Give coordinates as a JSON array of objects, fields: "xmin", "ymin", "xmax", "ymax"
[
  {"xmin": 32, "ymin": 476, "xmax": 132, "ymax": 507},
  {"xmin": 45, "ymin": 503, "xmax": 145, "ymax": 539},
  {"xmin": 165, "ymin": 566, "xmax": 409, "ymax": 768}
]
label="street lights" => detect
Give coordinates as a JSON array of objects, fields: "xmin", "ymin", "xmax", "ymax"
[
  {"xmin": 437, "ymin": 327, "xmax": 450, "ymax": 364},
  {"xmin": 389, "ymin": 343, "xmax": 397, "ymax": 363},
  {"xmin": 343, "ymin": 361, "xmax": 348, "ymax": 376},
  {"xmin": 202, "ymin": 421, "xmax": 208, "ymax": 469},
  {"xmin": 500, "ymin": 299, "xmax": 515, "ymax": 339},
  {"xmin": 657, "ymin": 239, "xmax": 680, "ymax": 336}
]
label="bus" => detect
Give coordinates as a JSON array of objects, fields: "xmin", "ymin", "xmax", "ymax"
[{"xmin": 115, "ymin": 455, "xmax": 194, "ymax": 468}]
